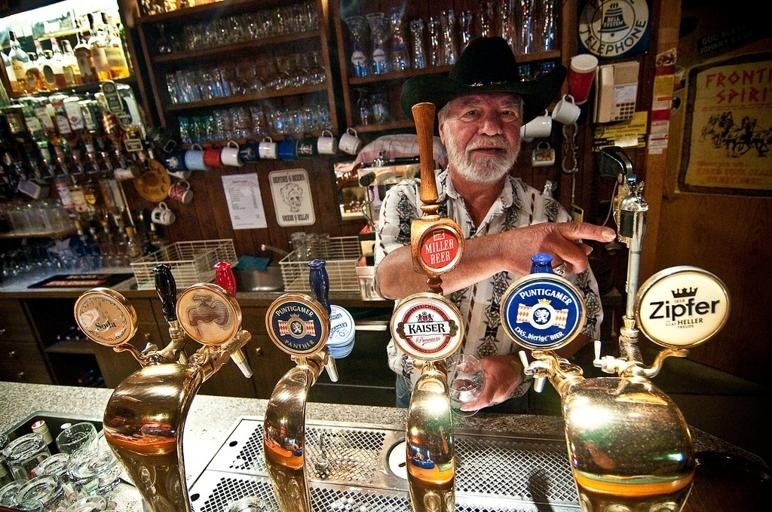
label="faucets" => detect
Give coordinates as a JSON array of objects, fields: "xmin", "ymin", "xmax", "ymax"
[{"xmin": 260, "ymin": 241, "xmax": 294, "ymax": 261}]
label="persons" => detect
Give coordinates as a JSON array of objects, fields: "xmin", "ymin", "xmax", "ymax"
[{"xmin": 374, "ymin": 37, "xmax": 618, "ymax": 411}]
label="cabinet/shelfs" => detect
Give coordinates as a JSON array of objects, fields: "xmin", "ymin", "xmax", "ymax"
[
  {"xmin": 2, "ymin": 296, "xmax": 59, "ymax": 382},
  {"xmin": 92, "ymin": 300, "xmax": 297, "ymax": 399},
  {"xmin": 136, "ymin": 0, "xmax": 571, "ymax": 150}
]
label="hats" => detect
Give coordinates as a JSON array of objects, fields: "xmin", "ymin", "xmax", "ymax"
[{"xmin": 401, "ymin": 36, "xmax": 565, "ymax": 118}]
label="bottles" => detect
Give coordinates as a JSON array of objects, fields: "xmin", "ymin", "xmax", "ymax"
[
  {"xmin": 32, "ymin": 36, "xmax": 64, "ymax": 91},
  {"xmin": 79, "ymin": 100, "xmax": 109, "ymax": 157},
  {"xmin": 69, "ymin": 175, "xmax": 93, "ymax": 224},
  {"xmin": 20, "ymin": 98, "xmax": 51, "ymax": 163},
  {"xmin": 101, "ymin": 13, "xmax": 130, "ymax": 80},
  {"xmin": 33, "ymin": 101, "xmax": 65, "ymax": 162},
  {"xmin": 126, "ymin": 227, "xmax": 141, "ymax": 263},
  {"xmin": 61, "ymin": 423, "xmax": 71, "ymax": 432},
  {"xmin": 32, "ymin": 420, "xmax": 59, "ymax": 455},
  {"xmin": 9, "ymin": 31, "xmax": 33, "ymax": 94},
  {"xmin": 88, "ymin": 13, "xmax": 113, "ymax": 81},
  {"xmin": 0, "ymin": 465, "xmax": 14, "ymax": 487},
  {"xmin": 74, "ymin": 19, "xmax": 99, "ymax": 82},
  {"xmin": 62, "ymin": 40, "xmax": 83, "ymax": 87},
  {"xmin": 117, "ymin": 216, "xmax": 134, "ymax": 253},
  {"xmin": 74, "ymin": 221, "xmax": 100, "ymax": 254},
  {"xmin": 48, "ymin": 94, "xmax": 80, "ymax": 160},
  {"xmin": 83, "ymin": 176, "xmax": 105, "ymax": 221},
  {"xmin": 101, "ymin": 221, "xmax": 117, "ymax": 253},
  {"xmin": 43, "ymin": 50, "xmax": 54, "ymax": 60},
  {"xmin": 2, "ymin": 141, "xmax": 24, "ymax": 173},
  {"xmin": 25, "ymin": 52, "xmax": 36, "ymax": 61},
  {"xmin": 50, "ymin": 37, "xmax": 62, "ymax": 60},
  {"xmin": 96, "ymin": 93, "xmax": 122, "ymax": 155},
  {"xmin": 64, "ymin": 96, "xmax": 94, "ymax": 157},
  {"xmin": 56, "ymin": 177, "xmax": 74, "ymax": 215},
  {"xmin": 5, "ymin": 107, "xmax": 40, "ymax": 167},
  {"xmin": 106, "ymin": 84, "xmax": 143, "ymax": 138},
  {"xmin": 118, "ymin": 10, "xmax": 135, "ymax": 77}
]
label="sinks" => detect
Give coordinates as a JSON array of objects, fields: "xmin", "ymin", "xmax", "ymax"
[{"xmin": 212, "ymin": 263, "xmax": 284, "ymax": 293}]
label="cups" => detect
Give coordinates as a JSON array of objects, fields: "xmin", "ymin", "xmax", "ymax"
[
  {"xmin": 0, "ymin": 422, "xmax": 123, "ymax": 511},
  {"xmin": 317, "ymin": 129, "xmax": 338, "ymax": 154},
  {"xmin": 264, "ymin": 98, "xmax": 333, "ymax": 136},
  {"xmin": 570, "ymin": 54, "xmax": 598, "ymax": 106},
  {"xmin": 276, "ymin": 134, "xmax": 297, "ymax": 161},
  {"xmin": 220, "ymin": 140, "xmax": 245, "ymax": 167},
  {"xmin": 289, "ymin": 232, "xmax": 306, "ymax": 261},
  {"xmin": 524, "ymin": 107, "xmax": 553, "ymax": 138},
  {"xmin": 446, "ymin": 353, "xmax": 486, "ymax": 418},
  {"xmin": 551, "ymin": 94, "xmax": 581, "ymax": 126},
  {"xmin": 162, "ymin": 148, "xmax": 185, "ymax": 173},
  {"xmin": 0, "ymin": 236, "xmax": 150, "ymax": 287},
  {"xmin": 151, "ymin": 201, "xmax": 175, "ymax": 226},
  {"xmin": 258, "ymin": 137, "xmax": 276, "ymax": 160},
  {"xmin": 531, "ymin": 141, "xmax": 556, "ymax": 167},
  {"xmin": 295, "ymin": 133, "xmax": 318, "ymax": 159},
  {"xmin": 165, "ymin": 167, "xmax": 192, "ymax": 180},
  {"xmin": 8, "ymin": 199, "xmax": 72, "ymax": 231},
  {"xmin": 176, "ymin": 103, "xmax": 266, "ymax": 140},
  {"xmin": 163, "ymin": 51, "xmax": 324, "ymax": 104},
  {"xmin": 144, "ymin": 2, "xmax": 320, "ymax": 54},
  {"xmin": 338, "ymin": 128, "xmax": 363, "ymax": 156},
  {"xmin": 157, "ymin": 129, "xmax": 177, "ymax": 153},
  {"xmin": 203, "ymin": 142, "xmax": 224, "ymax": 168},
  {"xmin": 167, "ymin": 180, "xmax": 194, "ymax": 204},
  {"xmin": 184, "ymin": 143, "xmax": 209, "ymax": 171},
  {"xmin": 302, "ymin": 234, "xmax": 330, "ymax": 262},
  {"xmin": 239, "ymin": 139, "xmax": 260, "ymax": 164}
]
